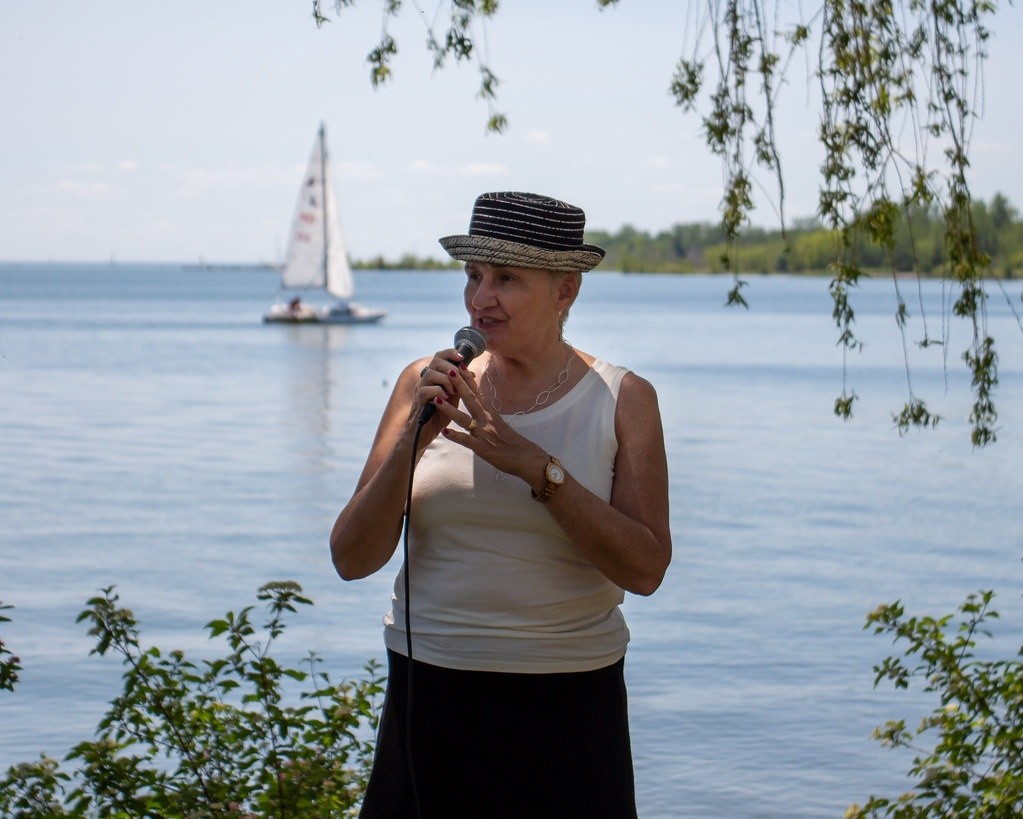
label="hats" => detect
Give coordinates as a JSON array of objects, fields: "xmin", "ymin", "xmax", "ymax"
[{"xmin": 439, "ymin": 192, "xmax": 606, "ymax": 272}]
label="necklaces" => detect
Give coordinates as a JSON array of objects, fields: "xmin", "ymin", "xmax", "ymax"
[{"xmin": 483, "ymin": 338, "xmax": 576, "ymax": 484}]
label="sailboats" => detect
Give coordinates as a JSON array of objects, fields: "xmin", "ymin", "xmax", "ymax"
[{"xmin": 263, "ymin": 121, "xmax": 387, "ymax": 324}]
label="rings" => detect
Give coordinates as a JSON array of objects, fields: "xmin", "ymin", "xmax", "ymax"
[
  {"xmin": 464, "ymin": 419, "xmax": 477, "ymax": 432},
  {"xmin": 420, "ymin": 366, "xmax": 428, "ymax": 377}
]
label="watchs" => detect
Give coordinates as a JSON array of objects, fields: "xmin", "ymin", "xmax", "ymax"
[{"xmin": 530, "ymin": 454, "xmax": 565, "ymax": 500}]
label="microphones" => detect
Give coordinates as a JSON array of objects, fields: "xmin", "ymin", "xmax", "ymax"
[{"xmin": 418, "ymin": 326, "xmax": 487, "ymax": 425}]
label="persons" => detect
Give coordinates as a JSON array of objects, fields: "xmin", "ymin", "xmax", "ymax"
[
  {"xmin": 290, "ymin": 297, "xmax": 300, "ymax": 309},
  {"xmin": 329, "ymin": 190, "xmax": 674, "ymax": 819}
]
